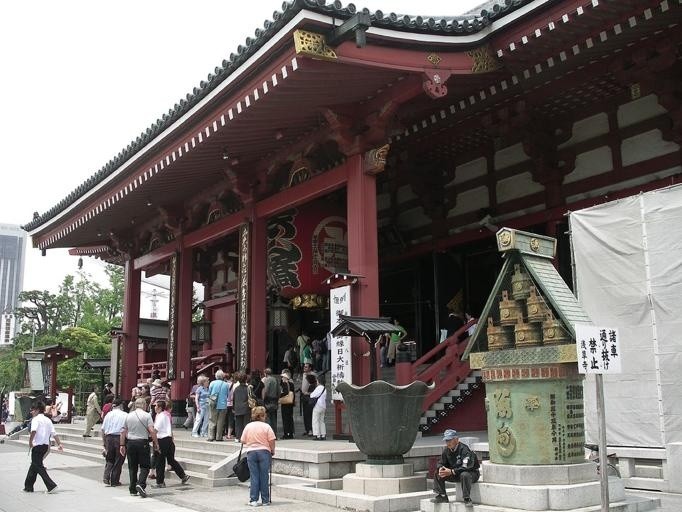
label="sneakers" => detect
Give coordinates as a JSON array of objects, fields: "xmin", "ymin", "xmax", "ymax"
[
  {"xmin": 280, "ymin": 432, "xmax": 293, "ymax": 440},
  {"xmin": 151, "ymin": 481, "xmax": 166, "ymax": 488},
  {"xmin": 135, "ymin": 484, "xmax": 147, "ymax": 497},
  {"xmin": 431, "ymin": 495, "xmax": 449, "ymax": 502},
  {"xmin": 148, "ymin": 475, "xmax": 157, "ymax": 479},
  {"xmin": 312, "ymin": 435, "xmax": 326, "ymax": 440},
  {"xmin": 44, "ymin": 487, "xmax": 58, "ymax": 494},
  {"xmin": 103, "ymin": 473, "xmax": 121, "ymax": 487},
  {"xmin": 464, "ymin": 497, "xmax": 473, "ymax": 507},
  {"xmin": 182, "ymin": 474, "xmax": 190, "ymax": 485},
  {"xmin": 248, "ymin": 500, "xmax": 258, "ymax": 507}
]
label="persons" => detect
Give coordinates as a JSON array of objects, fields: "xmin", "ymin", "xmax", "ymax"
[
  {"xmin": 151, "ymin": 401, "xmax": 191, "ymax": 488},
  {"xmin": 21, "ymin": 401, "xmax": 64, "ymax": 494},
  {"xmin": 128, "ymin": 369, "xmax": 171, "ymax": 419},
  {"xmin": 100, "ymin": 397, "xmax": 129, "ymax": 487},
  {"xmin": 183, "ymin": 368, "xmax": 264, "ymax": 442},
  {"xmin": 374, "ymin": 319, "xmax": 408, "ymax": 368},
  {"xmin": 241, "ymin": 405, "xmax": 277, "ymax": 506},
  {"xmin": 82, "ymin": 382, "xmax": 114, "ymax": 437},
  {"xmin": 119, "ymin": 397, "xmax": 161, "ymax": 497},
  {"xmin": 148, "ymin": 399, "xmax": 175, "ymax": 479},
  {"xmin": 444, "ymin": 309, "xmax": 478, "ymax": 343},
  {"xmin": 431, "ymin": 428, "xmax": 480, "ymax": 508},
  {"xmin": 255, "ymin": 330, "xmax": 331, "ymax": 442}
]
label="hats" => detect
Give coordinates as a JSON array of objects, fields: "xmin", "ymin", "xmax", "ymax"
[
  {"xmin": 111, "ymin": 398, "xmax": 124, "ymax": 406},
  {"xmin": 152, "ymin": 378, "xmax": 162, "ymax": 388},
  {"xmin": 443, "ymin": 430, "xmax": 456, "ymax": 440},
  {"xmin": 280, "ymin": 372, "xmax": 291, "ymax": 379}
]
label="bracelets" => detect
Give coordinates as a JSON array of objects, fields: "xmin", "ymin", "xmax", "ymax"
[{"xmin": 120, "ymin": 444, "xmax": 126, "ymax": 446}]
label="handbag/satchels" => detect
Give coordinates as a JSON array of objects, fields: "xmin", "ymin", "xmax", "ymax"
[
  {"xmin": 306, "ymin": 398, "xmax": 316, "ymax": 408},
  {"xmin": 248, "ymin": 396, "xmax": 256, "ymax": 408},
  {"xmin": 210, "ymin": 393, "xmax": 218, "ymax": 409},
  {"xmin": 233, "ymin": 457, "xmax": 250, "ymax": 482},
  {"xmin": 278, "ymin": 392, "xmax": 293, "ymax": 405}
]
